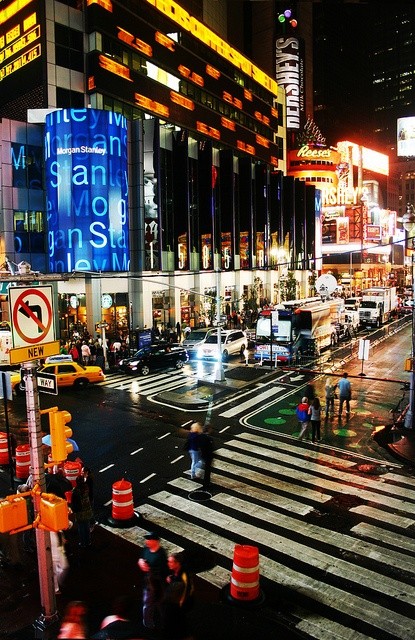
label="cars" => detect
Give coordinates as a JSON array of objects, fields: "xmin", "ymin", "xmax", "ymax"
[
  {"xmin": 244, "ymin": 319, "xmax": 257, "ymax": 342},
  {"xmin": 118, "ymin": 342, "xmax": 189, "ymax": 375},
  {"xmin": 0, "ymin": 364, "xmax": 23, "ymax": 393},
  {"xmin": 10, "ymin": 361, "xmax": 106, "ymax": 395}
]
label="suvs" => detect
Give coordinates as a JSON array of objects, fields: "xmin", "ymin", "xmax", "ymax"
[
  {"xmin": 196, "ymin": 329, "xmax": 248, "ymax": 361},
  {"xmin": 183, "ymin": 327, "xmax": 224, "ymax": 358}
]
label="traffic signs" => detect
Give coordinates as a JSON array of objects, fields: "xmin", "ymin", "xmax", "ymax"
[{"xmin": 36, "ymin": 372, "xmax": 58, "ymax": 395}]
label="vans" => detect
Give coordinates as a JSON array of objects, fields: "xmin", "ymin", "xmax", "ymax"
[{"xmin": 346, "ymin": 295, "xmax": 363, "ymax": 312}]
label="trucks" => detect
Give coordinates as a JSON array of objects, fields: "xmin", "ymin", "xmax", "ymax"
[
  {"xmin": 253, "ymin": 297, "xmax": 345, "ymax": 364},
  {"xmin": 359, "ymin": 286, "xmax": 397, "ymax": 327}
]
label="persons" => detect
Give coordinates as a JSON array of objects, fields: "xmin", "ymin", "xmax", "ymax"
[
  {"xmin": 175, "ymin": 321, "xmax": 182, "ymax": 340},
  {"xmin": 166, "ymin": 551, "xmax": 195, "ymax": 609},
  {"xmin": 45, "ymin": 468, "xmax": 73, "ymax": 500},
  {"xmin": 183, "ymin": 421, "xmax": 204, "ymax": 478},
  {"xmin": 308, "ymin": 396, "xmax": 323, "ymax": 442},
  {"xmin": 66, "ymin": 334, "xmax": 128, "ymax": 364},
  {"xmin": 135, "ymin": 530, "xmax": 167, "ymax": 625},
  {"xmin": 80, "ymin": 466, "xmax": 93, "ymax": 504},
  {"xmin": 16, "ymin": 466, "xmax": 35, "ymax": 495},
  {"xmin": 230, "ymin": 301, "xmax": 278, "ymax": 327},
  {"xmin": 295, "ymin": 395, "xmax": 311, "ymax": 442},
  {"xmin": 56, "ymin": 600, "xmax": 85, "ymax": 639},
  {"xmin": 336, "ymin": 373, "xmax": 353, "ymax": 415},
  {"xmin": 165, "ymin": 325, "xmax": 177, "ymax": 342},
  {"xmin": 50, "ymin": 520, "xmax": 73, "ymax": 595},
  {"xmin": 200, "ymin": 426, "xmax": 218, "ymax": 484},
  {"xmin": 70, "ymin": 472, "xmax": 97, "ymax": 544},
  {"xmin": 322, "ymin": 376, "xmax": 336, "ymax": 418}
]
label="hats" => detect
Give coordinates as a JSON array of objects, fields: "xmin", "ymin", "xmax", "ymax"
[{"xmin": 143, "ymin": 531, "xmax": 159, "ymax": 540}]
